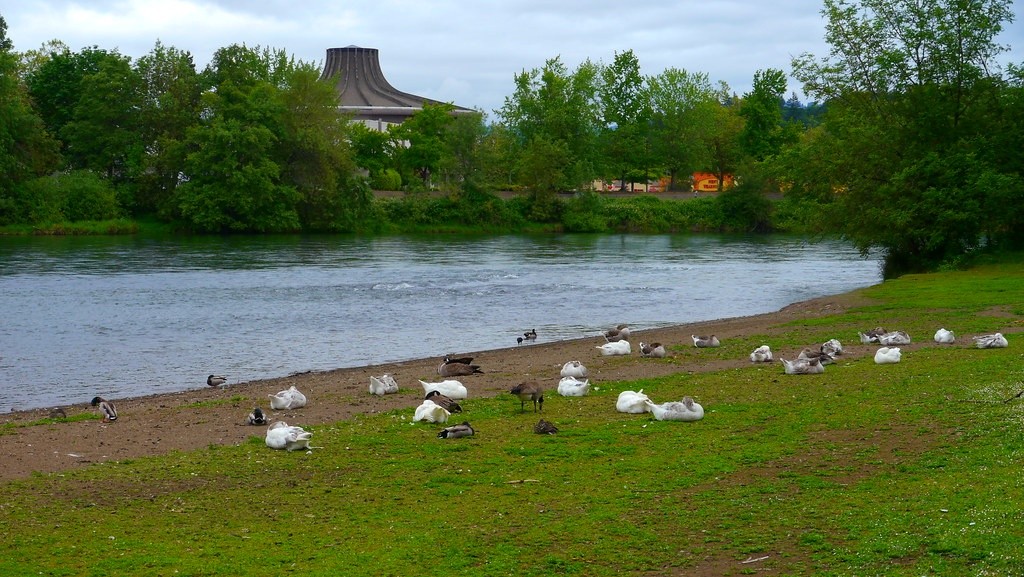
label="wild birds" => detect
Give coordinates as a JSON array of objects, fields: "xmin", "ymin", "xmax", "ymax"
[{"xmin": 47, "ymin": 324, "xmax": 1009, "ymax": 454}]
